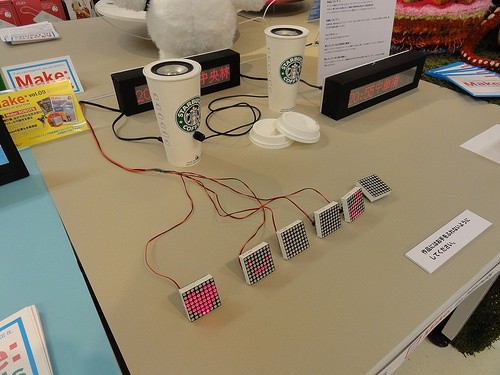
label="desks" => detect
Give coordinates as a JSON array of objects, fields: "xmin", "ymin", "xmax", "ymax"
[{"xmin": 0, "ymin": 0, "xmax": 500, "ymax": 375}]
